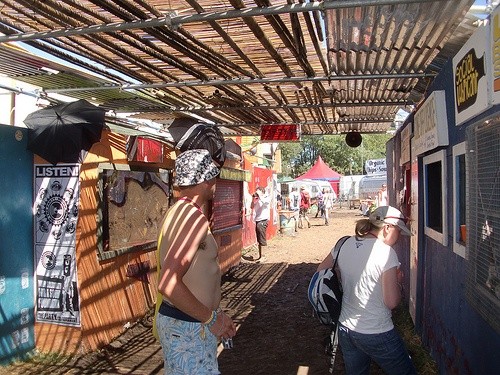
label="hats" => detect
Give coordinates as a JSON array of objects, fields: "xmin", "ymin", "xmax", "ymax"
[
  {"xmin": 173, "ymin": 149, "xmax": 222, "ymax": 186},
  {"xmin": 369, "ymin": 206, "xmax": 414, "ymax": 236}
]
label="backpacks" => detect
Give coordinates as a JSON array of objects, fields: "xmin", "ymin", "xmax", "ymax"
[{"xmin": 308, "ymin": 236, "xmax": 351, "ymax": 327}]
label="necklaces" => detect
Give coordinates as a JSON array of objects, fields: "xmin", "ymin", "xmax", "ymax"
[
  {"xmin": 177, "ymin": 197, "xmax": 203, "ymax": 214},
  {"xmin": 368, "ymin": 232, "xmax": 378, "ymax": 238}
]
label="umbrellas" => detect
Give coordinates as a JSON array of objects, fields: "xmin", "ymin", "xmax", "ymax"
[
  {"xmin": 167, "ymin": 117, "xmax": 227, "ymax": 170},
  {"xmin": 22, "ymin": 99, "xmax": 106, "ymax": 166}
]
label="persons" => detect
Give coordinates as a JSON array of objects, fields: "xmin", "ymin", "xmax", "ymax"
[
  {"xmin": 318, "ymin": 205, "xmax": 414, "ymax": 375},
  {"xmin": 315, "ymin": 186, "xmax": 332, "ymax": 226},
  {"xmin": 155, "ymin": 148, "xmax": 236, "ymax": 375},
  {"xmin": 378, "ymin": 184, "xmax": 388, "ymax": 206},
  {"xmin": 250, "ymin": 186, "xmax": 270, "ymax": 262},
  {"xmin": 289, "ymin": 184, "xmax": 311, "ymax": 232}
]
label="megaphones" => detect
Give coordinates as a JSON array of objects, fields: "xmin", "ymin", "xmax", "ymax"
[{"xmin": 345, "ymin": 132, "xmax": 362, "ymax": 147}]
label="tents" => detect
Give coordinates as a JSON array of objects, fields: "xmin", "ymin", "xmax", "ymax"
[{"xmin": 277, "ymin": 155, "xmax": 344, "ymax": 183}]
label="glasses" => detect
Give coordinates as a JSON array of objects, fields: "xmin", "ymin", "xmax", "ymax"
[{"xmin": 383, "ymin": 216, "xmax": 408, "ymax": 225}]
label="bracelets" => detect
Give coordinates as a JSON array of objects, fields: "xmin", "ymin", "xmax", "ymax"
[{"xmin": 204, "ymin": 310, "xmax": 218, "ymax": 328}]
